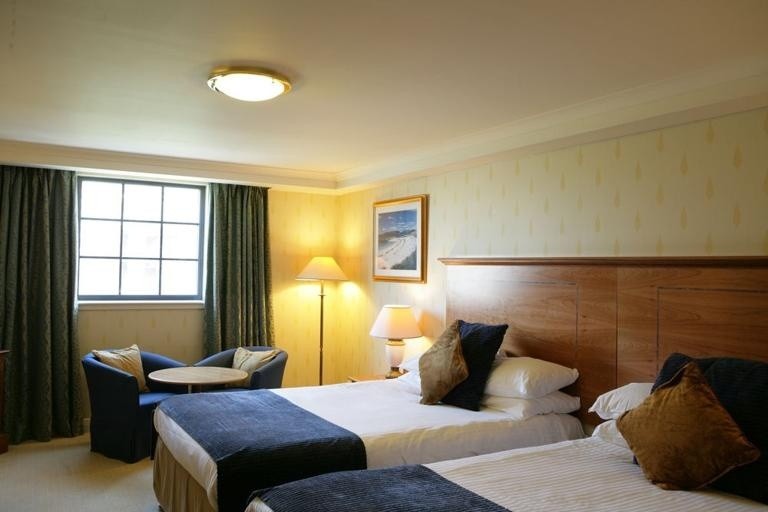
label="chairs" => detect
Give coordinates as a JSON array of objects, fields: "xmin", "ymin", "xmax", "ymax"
[
  {"xmin": 192, "ymin": 345, "xmax": 289, "ymax": 390},
  {"xmin": 81, "ymin": 348, "xmax": 190, "ymax": 464}
]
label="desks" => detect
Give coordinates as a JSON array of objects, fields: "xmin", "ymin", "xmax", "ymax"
[{"xmin": 148, "ymin": 365, "xmax": 248, "ymax": 393}]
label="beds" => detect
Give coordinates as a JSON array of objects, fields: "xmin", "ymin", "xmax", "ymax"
[
  {"xmin": 244, "ymin": 436, "xmax": 768, "ymax": 512},
  {"xmin": 152, "ymin": 379, "xmax": 586, "ymax": 512}
]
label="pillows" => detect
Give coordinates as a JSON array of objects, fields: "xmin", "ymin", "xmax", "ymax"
[
  {"xmin": 92, "ymin": 344, "xmax": 150, "ymax": 393},
  {"xmin": 396, "ymin": 319, "xmax": 582, "ymax": 422},
  {"xmin": 228, "ymin": 346, "xmax": 281, "ymax": 387},
  {"xmin": 585, "ymin": 353, "xmax": 768, "ymax": 504}
]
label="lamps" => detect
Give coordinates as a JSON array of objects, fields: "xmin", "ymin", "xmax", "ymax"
[
  {"xmin": 295, "ymin": 255, "xmax": 349, "ymax": 385},
  {"xmin": 205, "ymin": 65, "xmax": 293, "ymax": 102},
  {"xmin": 368, "ymin": 304, "xmax": 423, "ymax": 379}
]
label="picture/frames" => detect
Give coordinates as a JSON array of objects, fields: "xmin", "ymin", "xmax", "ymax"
[{"xmin": 371, "ymin": 193, "xmax": 428, "ymax": 283}]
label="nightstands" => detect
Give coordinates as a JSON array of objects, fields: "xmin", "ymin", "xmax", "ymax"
[{"xmin": 350, "ymin": 373, "xmax": 389, "ymax": 383}]
